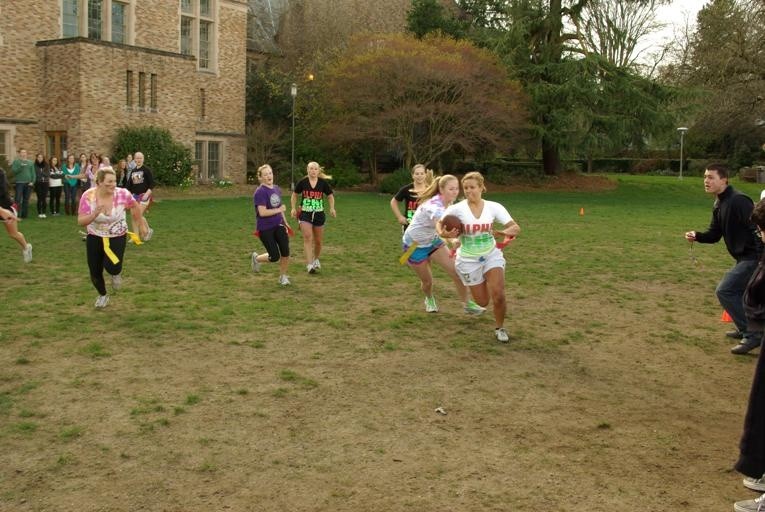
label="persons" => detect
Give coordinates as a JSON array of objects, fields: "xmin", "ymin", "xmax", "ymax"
[
  {"xmin": 733, "ymin": 189, "xmax": 765, "ymax": 512},
  {"xmin": 1, "ymin": 147, "xmax": 155, "ymax": 263},
  {"xmin": 76, "ymin": 166, "xmax": 150, "ymax": 308},
  {"xmin": 290, "ymin": 160, "xmax": 337, "ymax": 273},
  {"xmin": 251, "ymin": 163, "xmax": 291, "ymax": 287},
  {"xmin": 435, "ymin": 170, "xmax": 522, "ymax": 343},
  {"xmin": 685, "ymin": 163, "xmax": 761, "ymax": 355},
  {"xmin": 402, "ymin": 174, "xmax": 487, "ymax": 317},
  {"xmin": 389, "ymin": 163, "xmax": 427, "ymax": 232}
]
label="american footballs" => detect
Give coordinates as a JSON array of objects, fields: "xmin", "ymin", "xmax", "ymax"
[{"xmin": 441, "ymin": 215, "xmax": 463, "ymax": 236}]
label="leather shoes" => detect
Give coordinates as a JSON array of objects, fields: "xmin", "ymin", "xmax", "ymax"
[
  {"xmin": 727, "ymin": 329, "xmax": 743, "ymax": 339},
  {"xmin": 731, "ymin": 337, "xmax": 761, "ymax": 354}
]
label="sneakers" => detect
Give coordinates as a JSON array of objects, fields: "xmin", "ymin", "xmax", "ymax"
[
  {"xmin": 38, "ymin": 211, "xmax": 76, "ymax": 218},
  {"xmin": 127, "ymin": 239, "xmax": 134, "ymax": 244},
  {"xmin": 464, "ymin": 300, "xmax": 487, "ymax": 316},
  {"xmin": 252, "ymin": 252, "xmax": 320, "ymax": 286},
  {"xmin": 112, "ymin": 273, "xmax": 121, "ymax": 290},
  {"xmin": 144, "ymin": 228, "xmax": 153, "ymax": 241},
  {"xmin": 94, "ymin": 295, "xmax": 109, "ymax": 310},
  {"xmin": 425, "ymin": 297, "xmax": 437, "ymax": 312},
  {"xmin": 743, "ymin": 473, "xmax": 765, "ymax": 492},
  {"xmin": 23, "ymin": 243, "xmax": 32, "ymax": 262},
  {"xmin": 733, "ymin": 493, "xmax": 765, "ymax": 512},
  {"xmin": 495, "ymin": 328, "xmax": 508, "ymax": 342}
]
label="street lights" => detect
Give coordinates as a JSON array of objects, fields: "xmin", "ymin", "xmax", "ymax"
[
  {"xmin": 676, "ymin": 126, "xmax": 689, "ymax": 179},
  {"xmin": 289, "ymin": 81, "xmax": 298, "ymax": 189}
]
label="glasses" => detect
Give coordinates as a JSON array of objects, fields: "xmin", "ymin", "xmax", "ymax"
[{"xmin": 753, "ymin": 229, "xmax": 764, "ymax": 238}]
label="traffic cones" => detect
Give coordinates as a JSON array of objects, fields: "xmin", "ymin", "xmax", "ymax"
[
  {"xmin": 720, "ymin": 308, "xmax": 734, "ymax": 322},
  {"xmin": 578, "ymin": 207, "xmax": 586, "ymax": 215}
]
